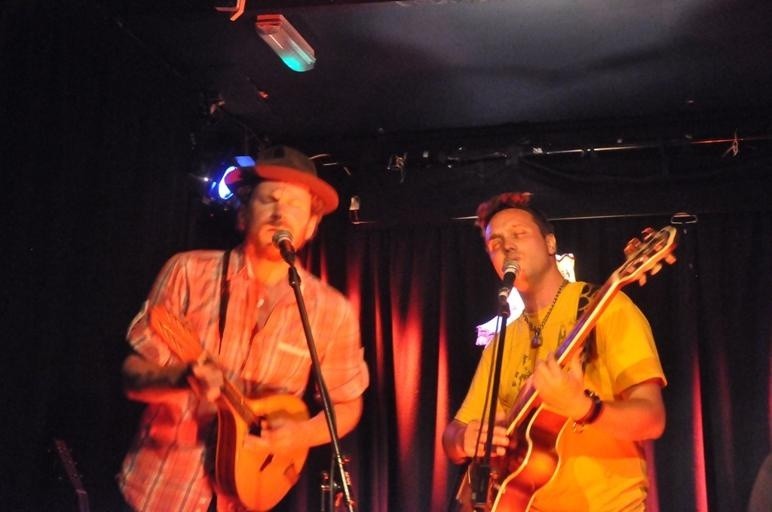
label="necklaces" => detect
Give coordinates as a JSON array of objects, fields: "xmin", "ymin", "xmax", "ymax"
[{"xmin": 521, "ymin": 279, "xmax": 569, "ymax": 348}]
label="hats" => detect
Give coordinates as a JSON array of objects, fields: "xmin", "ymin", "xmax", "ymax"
[{"xmin": 224, "ymin": 145, "xmax": 340, "ymax": 216}]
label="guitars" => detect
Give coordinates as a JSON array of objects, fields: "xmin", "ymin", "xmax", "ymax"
[{"xmin": 456, "ymin": 225, "xmax": 678, "ymax": 512}]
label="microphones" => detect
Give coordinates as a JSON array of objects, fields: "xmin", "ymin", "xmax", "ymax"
[
  {"xmin": 498, "ymin": 260, "xmax": 521, "ymax": 306},
  {"xmin": 272, "ymin": 230, "xmax": 296, "ymax": 267}
]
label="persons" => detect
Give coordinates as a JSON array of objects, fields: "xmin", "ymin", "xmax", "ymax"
[
  {"xmin": 441, "ymin": 194, "xmax": 667, "ymax": 511},
  {"xmin": 118, "ymin": 144, "xmax": 368, "ymax": 512}
]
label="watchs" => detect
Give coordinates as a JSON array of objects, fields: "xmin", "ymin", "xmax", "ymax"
[{"xmin": 575, "ymin": 388, "xmax": 603, "ymax": 426}]
